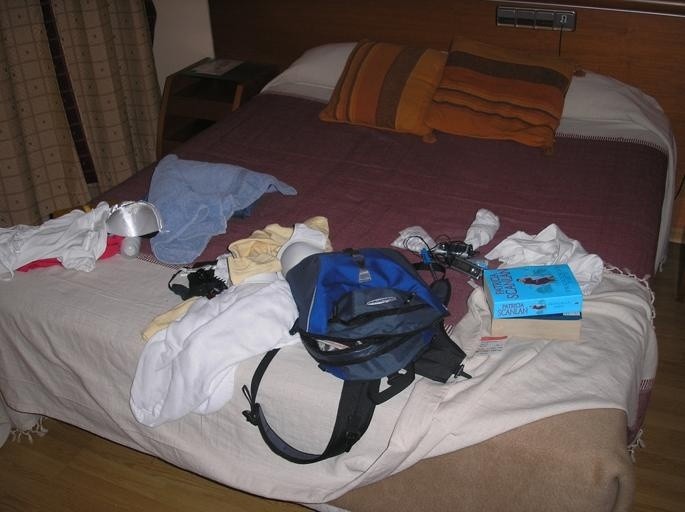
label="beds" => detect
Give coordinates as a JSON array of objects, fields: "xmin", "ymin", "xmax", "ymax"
[{"xmin": 0, "ymin": 92, "xmax": 684, "ymax": 511}]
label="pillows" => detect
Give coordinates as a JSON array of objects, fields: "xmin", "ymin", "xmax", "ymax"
[{"xmin": 317, "ymin": 36, "xmax": 575, "ymax": 158}]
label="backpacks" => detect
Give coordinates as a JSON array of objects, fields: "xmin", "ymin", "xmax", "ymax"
[{"xmin": 242, "ymin": 247, "xmax": 467, "ymax": 465}]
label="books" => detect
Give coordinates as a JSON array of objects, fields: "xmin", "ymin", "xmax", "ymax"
[
  {"xmin": 483, "ymin": 263, "xmax": 583, "ymax": 319},
  {"xmin": 482, "ymin": 306, "xmax": 582, "ymax": 341}
]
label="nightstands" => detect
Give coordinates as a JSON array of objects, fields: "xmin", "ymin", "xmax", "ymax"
[{"xmin": 155, "ymin": 57, "xmax": 279, "ymax": 162}]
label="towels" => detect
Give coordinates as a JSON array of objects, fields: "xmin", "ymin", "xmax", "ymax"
[{"xmin": 135, "ymin": 149, "xmax": 298, "ymax": 267}]
